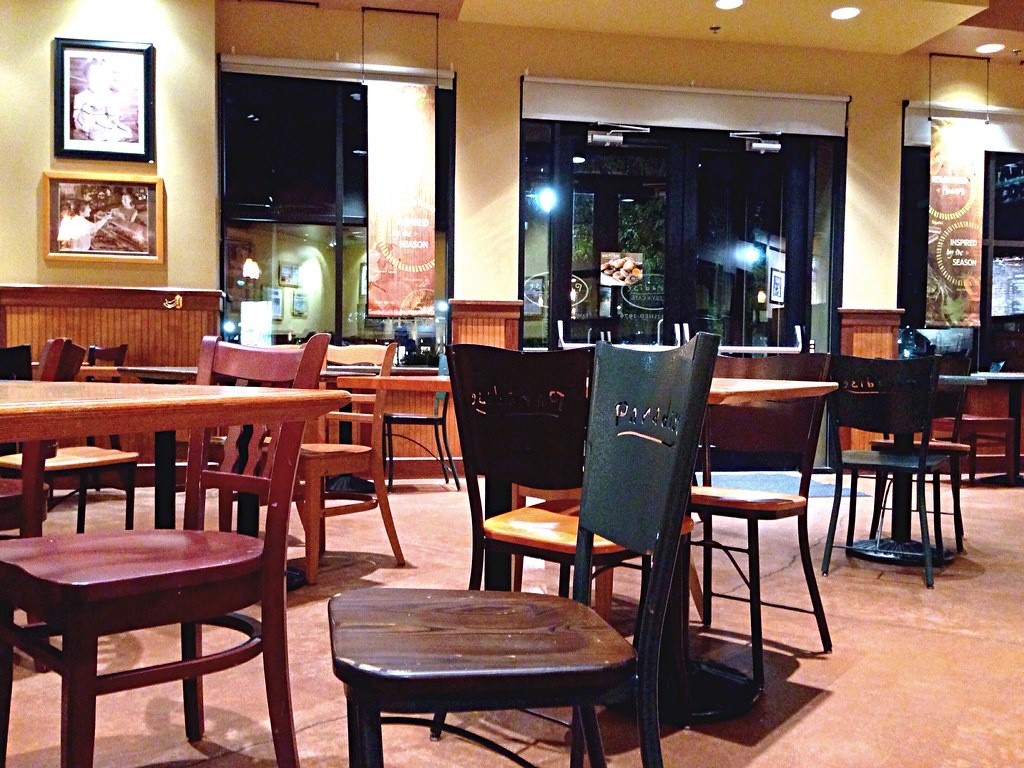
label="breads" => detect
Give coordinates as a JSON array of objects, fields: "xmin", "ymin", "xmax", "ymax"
[{"xmin": 601, "ymin": 258, "xmax": 641, "ymax": 285}]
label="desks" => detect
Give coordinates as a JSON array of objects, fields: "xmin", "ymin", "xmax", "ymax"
[
  {"xmin": 339, "ymin": 375, "xmax": 841, "ymax": 657},
  {"xmin": 971, "ymin": 372, "xmax": 1024, "ymax": 492},
  {"xmin": 0, "ymin": 379, "xmax": 353, "ymax": 768},
  {"xmin": 849, "ymin": 376, "xmax": 985, "ymax": 566}
]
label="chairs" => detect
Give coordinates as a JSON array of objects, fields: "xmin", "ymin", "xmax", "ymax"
[{"xmin": 0, "ymin": 316, "xmax": 1024, "ymax": 768}]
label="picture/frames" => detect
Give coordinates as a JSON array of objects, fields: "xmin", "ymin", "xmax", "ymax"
[
  {"xmin": 260, "ymin": 283, "xmax": 284, "ymax": 319},
  {"xmin": 359, "ymin": 262, "xmax": 368, "ymax": 298},
  {"xmin": 770, "ymin": 267, "xmax": 785, "ymax": 305},
  {"xmin": 293, "ymin": 290, "xmax": 308, "ymax": 317},
  {"xmin": 279, "ymin": 261, "xmax": 302, "ymax": 288},
  {"xmin": 52, "ymin": 38, "xmax": 156, "ymax": 162},
  {"xmin": 39, "ymin": 168, "xmax": 165, "ymax": 268},
  {"xmin": 523, "ymin": 275, "xmax": 545, "ymax": 321}
]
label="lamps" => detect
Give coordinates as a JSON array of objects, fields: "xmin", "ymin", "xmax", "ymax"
[
  {"xmin": 588, "ymin": 122, "xmax": 650, "ymax": 148},
  {"xmin": 728, "ymin": 130, "xmax": 782, "ymax": 154}
]
